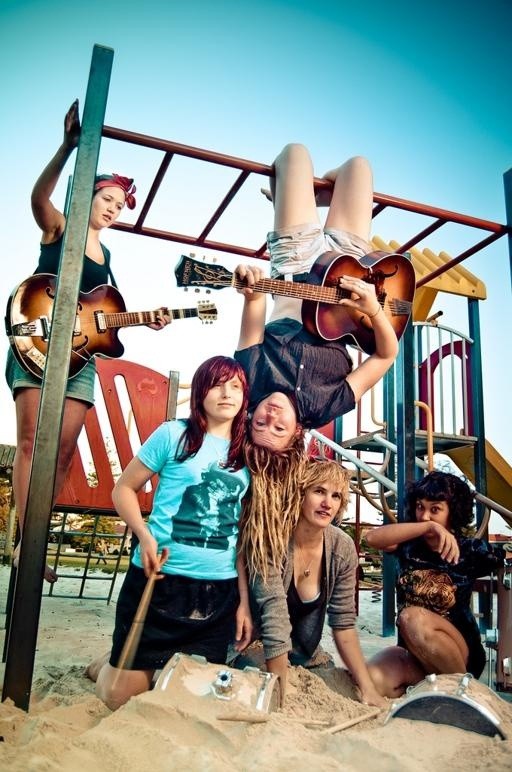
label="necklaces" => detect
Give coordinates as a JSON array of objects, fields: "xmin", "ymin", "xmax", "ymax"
[
  {"xmin": 294, "ymin": 533, "xmax": 324, "ymax": 579},
  {"xmin": 206, "ymin": 431, "xmax": 231, "ymax": 469}
]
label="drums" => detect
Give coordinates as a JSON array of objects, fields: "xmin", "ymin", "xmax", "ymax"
[
  {"xmin": 390, "ymin": 672, "xmax": 508, "ymax": 741},
  {"xmin": 153, "ymin": 652, "xmax": 284, "ymax": 713}
]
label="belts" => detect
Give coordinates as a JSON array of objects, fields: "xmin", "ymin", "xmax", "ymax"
[{"xmin": 275, "ymin": 272, "xmax": 309, "ymax": 282}]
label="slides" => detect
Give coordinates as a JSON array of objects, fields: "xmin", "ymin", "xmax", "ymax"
[{"xmin": 442, "ymin": 440, "xmax": 512, "ymax": 527}]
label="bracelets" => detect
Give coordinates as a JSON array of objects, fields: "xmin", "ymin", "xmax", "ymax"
[
  {"xmin": 369, "ymin": 303, "xmax": 382, "ymax": 319},
  {"xmin": 358, "ymin": 283, "xmax": 365, "ymax": 288}
]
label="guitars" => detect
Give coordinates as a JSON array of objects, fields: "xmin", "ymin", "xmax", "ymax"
[
  {"xmin": 5, "ymin": 273, "xmax": 217, "ymax": 384},
  {"xmin": 174, "ymin": 251, "xmax": 415, "ymax": 356}
]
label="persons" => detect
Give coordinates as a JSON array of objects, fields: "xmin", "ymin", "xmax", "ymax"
[
  {"xmin": 95, "ymin": 538, "xmax": 108, "ymax": 565},
  {"xmin": 363, "ymin": 470, "xmax": 511, "ymax": 701},
  {"xmin": 84, "ymin": 353, "xmax": 256, "ymax": 713},
  {"xmin": 230, "ymin": 142, "xmax": 403, "ymax": 588},
  {"xmin": 225, "ymin": 455, "xmax": 394, "ymax": 712},
  {"xmin": 4, "ymin": 98, "xmax": 175, "ymax": 586}
]
